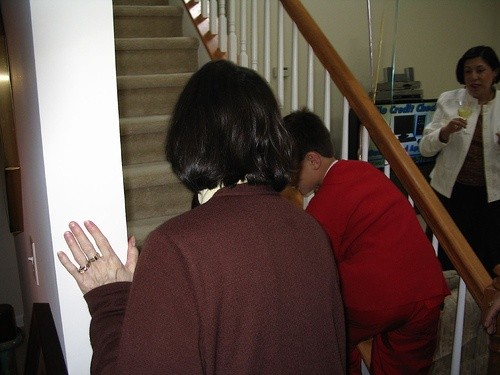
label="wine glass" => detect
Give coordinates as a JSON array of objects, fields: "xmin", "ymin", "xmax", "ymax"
[{"xmin": 455, "ymin": 99, "xmax": 475, "ymax": 136}]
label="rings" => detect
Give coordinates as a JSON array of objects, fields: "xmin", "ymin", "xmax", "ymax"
[
  {"xmin": 78, "ymin": 261, "xmax": 91, "ymax": 274},
  {"xmin": 88, "ymin": 253, "xmax": 100, "ymax": 262}
]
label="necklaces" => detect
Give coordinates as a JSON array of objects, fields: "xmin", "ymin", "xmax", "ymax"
[{"xmin": 315, "ymin": 157, "xmax": 349, "ymax": 184}]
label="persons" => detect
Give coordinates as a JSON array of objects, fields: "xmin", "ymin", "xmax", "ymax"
[
  {"xmin": 420, "ymin": 46, "xmax": 500, "ymax": 215},
  {"xmin": 482, "ymin": 261, "xmax": 499, "ymax": 340},
  {"xmin": 275, "ymin": 107, "xmax": 452, "ymax": 374},
  {"xmin": 56, "ymin": 59, "xmax": 349, "ymax": 374}
]
